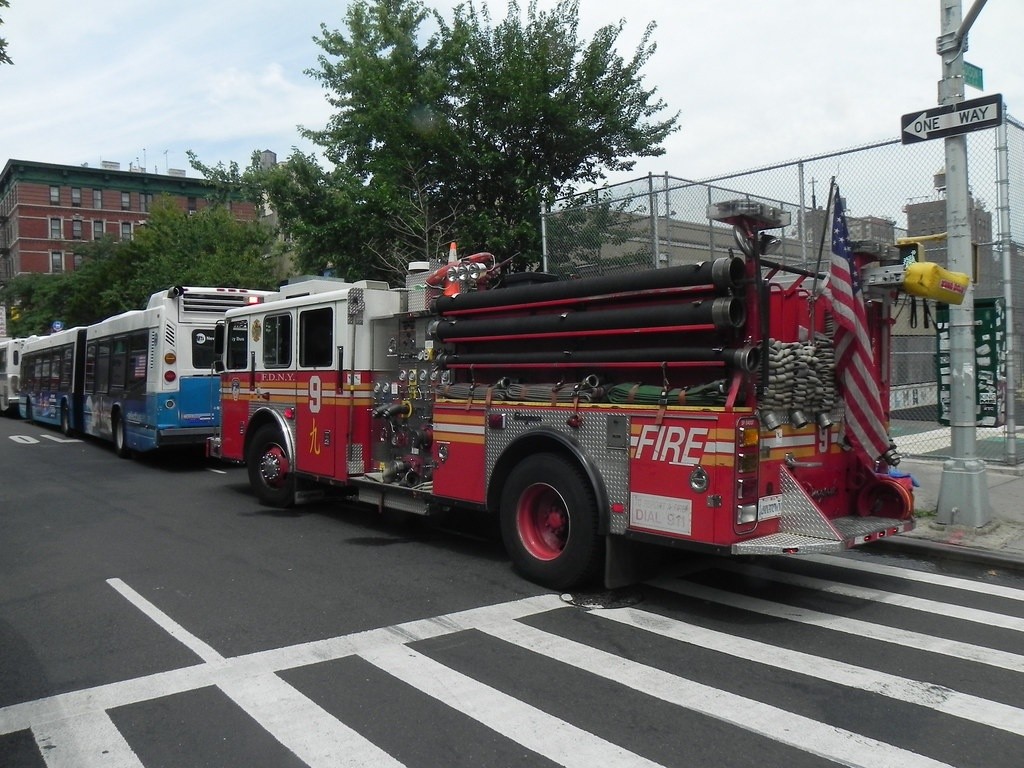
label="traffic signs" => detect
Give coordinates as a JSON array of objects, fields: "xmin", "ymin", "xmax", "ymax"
[{"xmin": 901, "ymin": 93, "xmax": 1003, "ymax": 147}]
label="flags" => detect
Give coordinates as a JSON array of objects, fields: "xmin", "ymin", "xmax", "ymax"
[{"xmin": 820, "ymin": 184, "xmax": 921, "ymax": 489}]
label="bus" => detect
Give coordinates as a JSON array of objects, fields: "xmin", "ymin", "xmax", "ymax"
[{"xmin": 0, "ymin": 286, "xmax": 279, "ymax": 463}]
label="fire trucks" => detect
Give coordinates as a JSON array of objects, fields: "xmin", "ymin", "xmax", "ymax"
[{"xmin": 212, "ymin": 175, "xmax": 972, "ymax": 596}]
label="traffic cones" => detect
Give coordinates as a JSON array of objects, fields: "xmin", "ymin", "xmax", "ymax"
[{"xmin": 444, "ymin": 241, "xmax": 459, "ymax": 297}]
